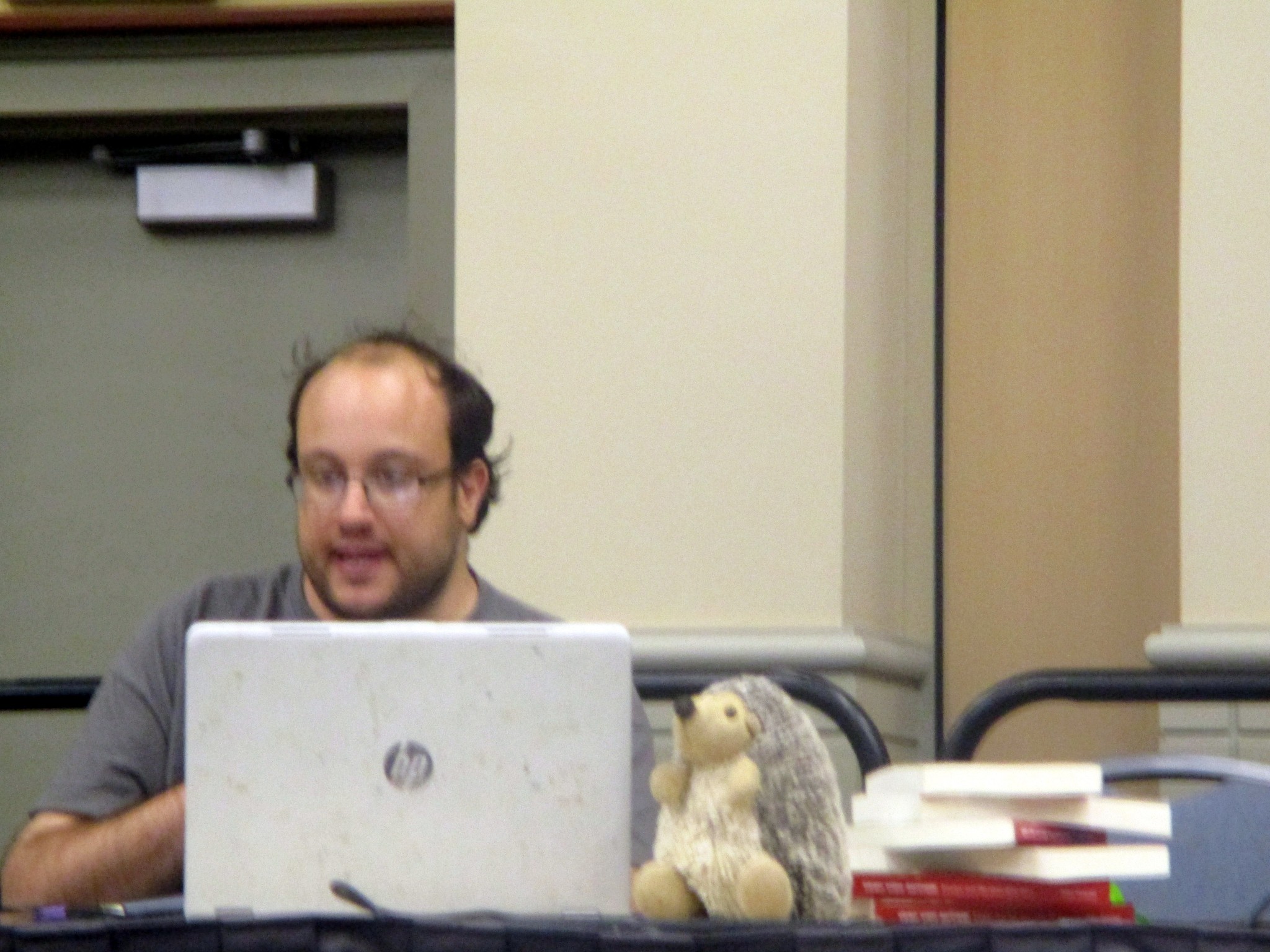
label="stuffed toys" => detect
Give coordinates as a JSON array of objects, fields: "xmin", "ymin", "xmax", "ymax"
[{"xmin": 636, "ymin": 688, "xmax": 790, "ymax": 930}]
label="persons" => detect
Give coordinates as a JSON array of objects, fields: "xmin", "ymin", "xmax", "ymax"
[{"xmin": 0, "ymin": 326, "xmax": 657, "ymax": 930}]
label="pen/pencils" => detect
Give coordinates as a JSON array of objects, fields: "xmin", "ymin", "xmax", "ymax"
[{"xmin": 35, "ymin": 904, "xmax": 101, "ymax": 918}]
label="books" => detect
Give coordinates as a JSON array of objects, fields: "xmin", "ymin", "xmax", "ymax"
[{"xmin": 844, "ymin": 750, "xmax": 1175, "ymax": 933}]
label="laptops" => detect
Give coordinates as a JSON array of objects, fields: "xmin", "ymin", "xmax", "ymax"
[{"xmin": 181, "ymin": 617, "xmax": 634, "ymax": 925}]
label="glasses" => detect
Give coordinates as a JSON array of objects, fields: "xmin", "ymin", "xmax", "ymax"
[{"xmin": 284, "ymin": 465, "xmax": 452, "ymax": 502}]
